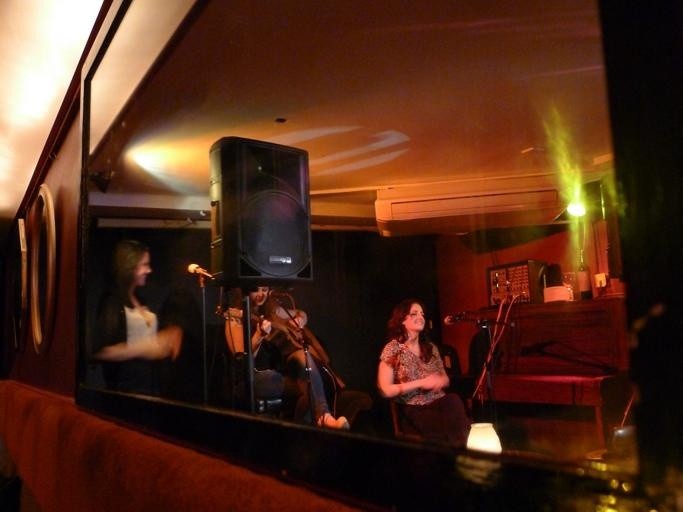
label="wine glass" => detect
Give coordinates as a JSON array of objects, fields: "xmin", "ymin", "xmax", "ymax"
[{"xmin": 561, "ymin": 263, "xmax": 577, "ymax": 301}]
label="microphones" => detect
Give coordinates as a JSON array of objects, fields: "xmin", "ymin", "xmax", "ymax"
[
  {"xmin": 269, "ymin": 289, "xmax": 287, "ymax": 298},
  {"xmin": 445, "ymin": 311, "xmax": 464, "ymax": 325},
  {"xmin": 187, "ymin": 264, "xmax": 216, "ymax": 282}
]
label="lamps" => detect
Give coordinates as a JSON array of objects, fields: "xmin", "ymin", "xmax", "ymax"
[{"xmin": 86, "ymin": 168, "xmax": 114, "ymax": 194}]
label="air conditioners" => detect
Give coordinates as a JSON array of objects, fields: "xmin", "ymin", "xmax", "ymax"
[{"xmin": 375, "ymin": 178, "xmax": 569, "ymax": 231}]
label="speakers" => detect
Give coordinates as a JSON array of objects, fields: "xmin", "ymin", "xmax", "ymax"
[{"xmin": 211, "ymin": 137, "xmax": 315, "ymax": 287}]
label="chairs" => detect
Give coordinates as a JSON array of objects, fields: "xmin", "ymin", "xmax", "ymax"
[
  {"xmin": 224, "ymin": 311, "xmax": 329, "ymax": 416},
  {"xmin": 388, "ymin": 395, "xmax": 427, "ymax": 442}
]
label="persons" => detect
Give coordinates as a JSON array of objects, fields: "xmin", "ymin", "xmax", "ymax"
[
  {"xmin": 223, "ymin": 283, "xmax": 352, "ymax": 431},
  {"xmin": 375, "ymin": 295, "xmax": 471, "ymax": 452},
  {"xmin": 92, "ymin": 233, "xmax": 184, "ymax": 396}
]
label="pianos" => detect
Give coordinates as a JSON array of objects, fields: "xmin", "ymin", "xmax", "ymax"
[{"xmin": 476, "ymin": 295, "xmax": 632, "ymax": 449}]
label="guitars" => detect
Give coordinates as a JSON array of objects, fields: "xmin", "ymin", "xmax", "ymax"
[{"xmin": 216, "ymin": 306, "xmax": 330, "ymax": 365}]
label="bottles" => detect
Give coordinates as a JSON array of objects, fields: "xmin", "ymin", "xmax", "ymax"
[
  {"xmin": 607, "ymin": 424, "xmax": 638, "ymax": 476},
  {"xmin": 577, "ymin": 248, "xmax": 591, "ymax": 298}
]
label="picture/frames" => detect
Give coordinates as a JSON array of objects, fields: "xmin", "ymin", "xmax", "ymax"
[{"xmin": 28, "ymin": 185, "xmax": 56, "ymax": 353}]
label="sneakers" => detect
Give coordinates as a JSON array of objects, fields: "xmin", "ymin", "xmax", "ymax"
[{"xmin": 318, "ymin": 412, "xmax": 349, "ymax": 429}]
